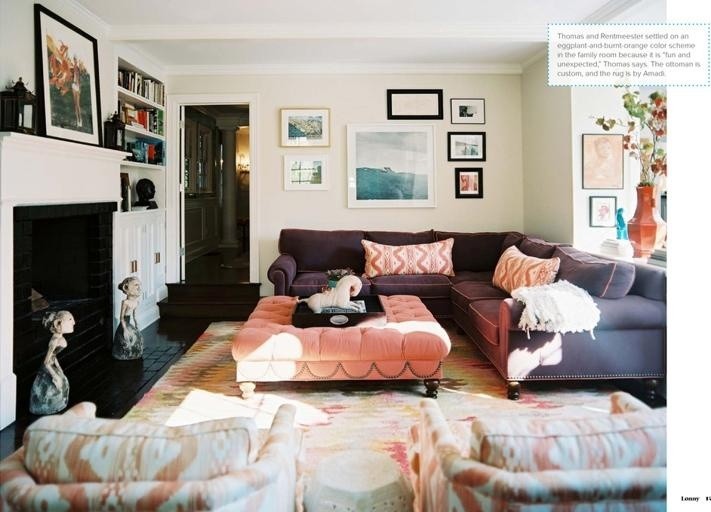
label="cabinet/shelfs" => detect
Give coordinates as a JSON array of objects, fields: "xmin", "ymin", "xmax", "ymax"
[
  {"xmin": 185, "ymin": 108, "xmax": 219, "ymax": 263},
  {"xmin": 154, "ymin": 212, "xmax": 168, "ymax": 303},
  {"xmin": 114, "ymin": 55, "xmax": 169, "ymax": 213},
  {"xmin": 113, "ymin": 215, "xmax": 161, "ymax": 331}
]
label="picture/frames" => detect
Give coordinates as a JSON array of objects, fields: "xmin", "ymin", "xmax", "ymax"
[
  {"xmin": 447, "ymin": 131, "xmax": 487, "ymax": 161},
  {"xmin": 346, "ymin": 122, "xmax": 438, "ymax": 209},
  {"xmin": 450, "ymin": 98, "xmax": 486, "ymax": 125},
  {"xmin": 284, "ymin": 155, "xmax": 330, "ymax": 192},
  {"xmin": 581, "ymin": 133, "xmax": 624, "ymax": 190},
  {"xmin": 279, "ymin": 108, "xmax": 333, "ymax": 148},
  {"xmin": 34, "ymin": 3, "xmax": 104, "ymax": 147},
  {"xmin": 455, "ymin": 167, "xmax": 484, "ymax": 200},
  {"xmin": 589, "ymin": 195, "xmax": 617, "ymax": 228},
  {"xmin": 387, "ymin": 89, "xmax": 444, "ymax": 121}
]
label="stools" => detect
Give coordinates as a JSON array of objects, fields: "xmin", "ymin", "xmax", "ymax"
[{"xmin": 302, "ymin": 449, "xmax": 415, "ymax": 511}]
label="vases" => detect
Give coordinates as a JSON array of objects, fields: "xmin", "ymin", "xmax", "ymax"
[
  {"xmin": 652, "ymin": 188, "xmax": 667, "ymax": 251},
  {"xmin": 628, "ymin": 187, "xmax": 666, "ymax": 257}
]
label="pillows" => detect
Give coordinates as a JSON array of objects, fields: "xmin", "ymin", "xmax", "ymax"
[
  {"xmin": 520, "ymin": 236, "xmax": 556, "ymax": 258},
  {"xmin": 361, "ymin": 238, "xmax": 456, "ymax": 279},
  {"xmin": 470, "ymin": 409, "xmax": 665, "ymax": 470},
  {"xmin": 552, "ymin": 247, "xmax": 637, "ymax": 299},
  {"xmin": 21, "ymin": 413, "xmax": 258, "ymax": 483},
  {"xmin": 492, "ymin": 245, "xmax": 561, "ymax": 293}
]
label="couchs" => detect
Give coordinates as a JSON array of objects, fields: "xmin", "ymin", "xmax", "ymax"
[
  {"xmin": 418, "ymin": 391, "xmax": 666, "ymax": 512},
  {"xmin": 437, "ymin": 230, "xmax": 667, "ymax": 406},
  {"xmin": 267, "ymin": 228, "xmax": 452, "ymax": 318},
  {"xmin": 0, "ymin": 401, "xmax": 299, "ymax": 512}
]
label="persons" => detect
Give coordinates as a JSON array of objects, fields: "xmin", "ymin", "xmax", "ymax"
[
  {"xmin": 113, "ymin": 276, "xmax": 144, "ymax": 361},
  {"xmin": 134, "ymin": 177, "xmax": 159, "ymax": 211},
  {"xmin": 588, "ymin": 137, "xmax": 621, "ymax": 186},
  {"xmin": 28, "ymin": 309, "xmax": 76, "ymax": 416},
  {"xmin": 68, "ymin": 56, "xmax": 83, "ymax": 132}
]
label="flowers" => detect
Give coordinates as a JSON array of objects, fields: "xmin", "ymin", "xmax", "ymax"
[
  {"xmin": 643, "ymin": 90, "xmax": 667, "ymax": 185},
  {"xmin": 592, "ymin": 87, "xmax": 655, "ymax": 188}
]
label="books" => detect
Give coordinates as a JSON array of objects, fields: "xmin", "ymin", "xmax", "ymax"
[
  {"xmin": 118, "ymin": 69, "xmax": 165, "ymax": 109},
  {"xmin": 119, "ymin": 99, "xmax": 165, "ymax": 136}
]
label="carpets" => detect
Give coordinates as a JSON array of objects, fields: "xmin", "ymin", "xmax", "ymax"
[{"xmin": 122, "ymin": 321, "xmax": 614, "ymax": 478}]
